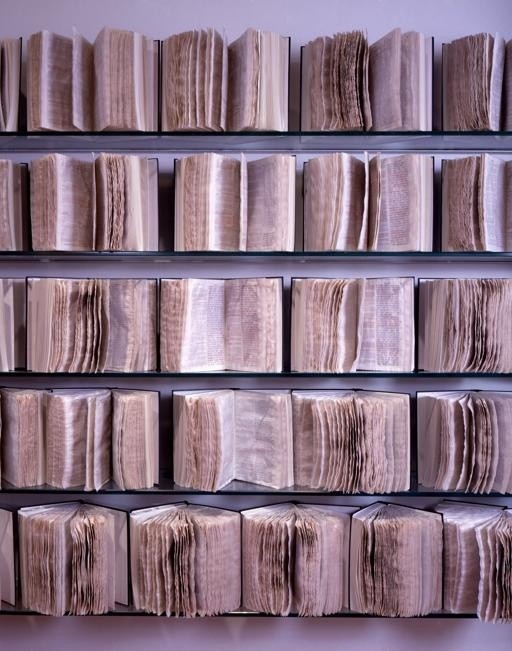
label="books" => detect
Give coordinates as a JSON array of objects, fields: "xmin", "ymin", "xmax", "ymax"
[
  {"xmin": 2, "ymin": 385, "xmax": 511, "ymax": 495},
  {"xmin": 1, "ymin": 275, "xmax": 512, "ymax": 377},
  {"xmin": 0, "ymin": 150, "xmax": 512, "ymax": 254},
  {"xmin": 1, "ymin": 501, "xmax": 511, "ymax": 626},
  {"xmin": 2, "ymin": 25, "xmax": 512, "ymax": 136}
]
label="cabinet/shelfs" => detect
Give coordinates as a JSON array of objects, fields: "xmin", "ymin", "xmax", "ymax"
[{"xmin": 0, "ymin": 132, "xmax": 512, "ymax": 618}]
